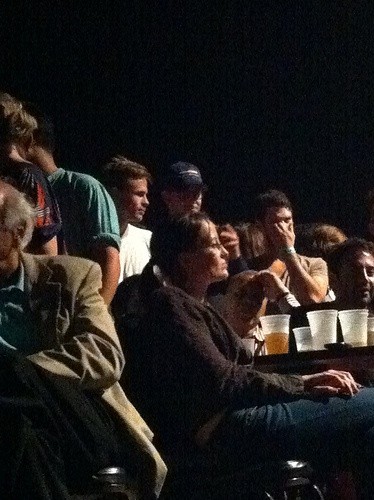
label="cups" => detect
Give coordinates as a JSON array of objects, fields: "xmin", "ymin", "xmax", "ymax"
[
  {"xmin": 368, "ymin": 317, "xmax": 374, "ymax": 346},
  {"xmin": 339, "ymin": 309, "xmax": 368, "ymax": 347},
  {"xmin": 306, "ymin": 310, "xmax": 338, "ymax": 350},
  {"xmin": 293, "ymin": 327, "xmax": 312, "ymax": 351},
  {"xmin": 259, "ymin": 315, "xmax": 290, "ymax": 354}
]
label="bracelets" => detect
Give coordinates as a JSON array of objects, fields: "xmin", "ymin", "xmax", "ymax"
[
  {"xmin": 277, "ymin": 246, "xmax": 297, "ymax": 259},
  {"xmin": 275, "ymin": 291, "xmax": 291, "ymax": 303}
]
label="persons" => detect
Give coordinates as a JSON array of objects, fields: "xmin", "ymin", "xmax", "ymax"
[{"xmin": 0, "ymin": 93, "xmax": 374, "ymax": 500}]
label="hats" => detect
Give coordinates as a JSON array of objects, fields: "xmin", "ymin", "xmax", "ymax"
[{"xmin": 164, "ymin": 162, "xmax": 206, "ymax": 191}]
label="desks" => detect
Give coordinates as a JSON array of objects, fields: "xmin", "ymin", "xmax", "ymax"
[{"xmin": 252, "ymin": 346, "xmax": 374, "ymax": 388}]
